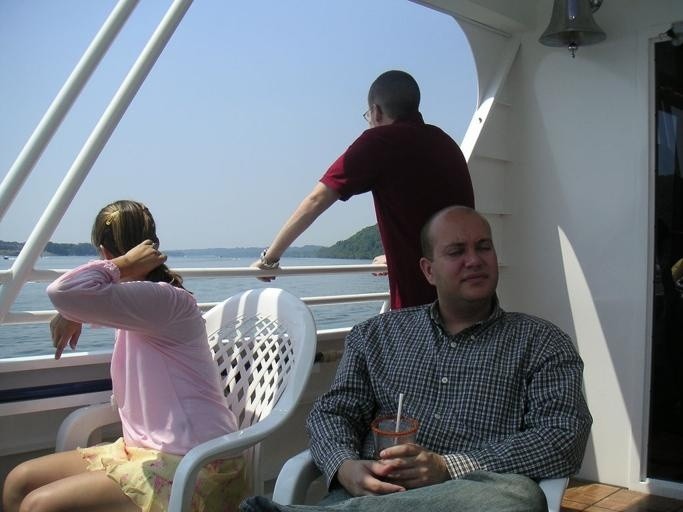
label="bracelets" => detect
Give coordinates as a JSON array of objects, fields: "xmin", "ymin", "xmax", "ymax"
[{"xmin": 260, "ymin": 247, "xmax": 280, "ymax": 270}]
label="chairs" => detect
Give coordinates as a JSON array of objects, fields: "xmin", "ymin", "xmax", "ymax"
[{"xmin": 54, "ymin": 285, "xmax": 319, "ymax": 512}]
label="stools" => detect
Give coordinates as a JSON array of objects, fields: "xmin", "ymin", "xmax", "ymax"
[{"xmin": 270, "ymin": 448, "xmax": 571, "ymax": 512}]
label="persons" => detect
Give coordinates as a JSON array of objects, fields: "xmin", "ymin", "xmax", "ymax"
[
  {"xmin": 0, "ymin": 198, "xmax": 248, "ymax": 511},
  {"xmin": 237, "ymin": 204, "xmax": 595, "ymax": 510},
  {"xmin": 249, "ymin": 68, "xmax": 475, "ymax": 314}
]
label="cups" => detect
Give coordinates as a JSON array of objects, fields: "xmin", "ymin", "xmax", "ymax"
[{"xmin": 369, "ymin": 414, "xmax": 419, "ymax": 461}]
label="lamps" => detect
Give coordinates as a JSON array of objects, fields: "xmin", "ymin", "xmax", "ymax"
[{"xmin": 536, "ymin": 0, "xmax": 606, "ymax": 58}]
